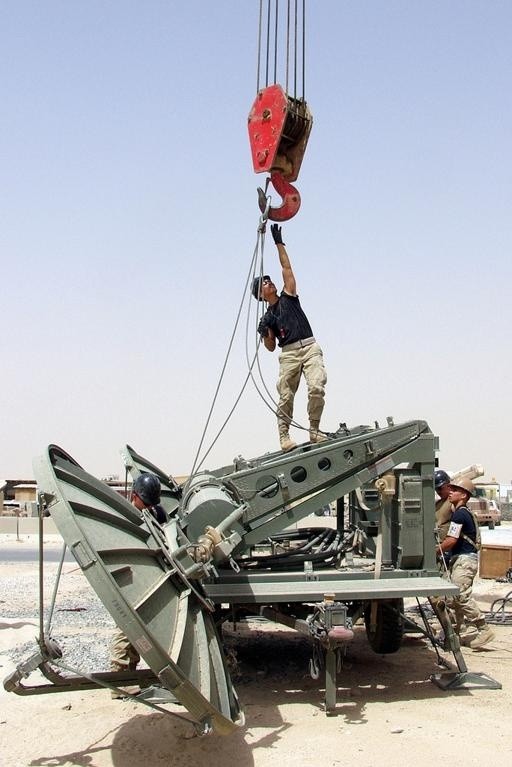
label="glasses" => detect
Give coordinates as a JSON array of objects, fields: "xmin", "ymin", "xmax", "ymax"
[{"xmin": 259, "ymin": 279, "xmax": 272, "ymax": 287}]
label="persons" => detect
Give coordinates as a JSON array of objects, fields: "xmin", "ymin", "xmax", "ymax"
[
  {"xmin": 110, "ymin": 472, "xmax": 161, "ymax": 699},
  {"xmin": 433, "ymin": 470, "xmax": 468, "ymax": 635},
  {"xmin": 437, "ymin": 476, "xmax": 496, "ymax": 649},
  {"xmin": 251, "ymin": 222, "xmax": 327, "ymax": 452}
]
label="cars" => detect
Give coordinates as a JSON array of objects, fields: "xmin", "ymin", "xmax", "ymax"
[{"xmin": 466, "ymin": 496, "xmax": 502, "ymax": 529}]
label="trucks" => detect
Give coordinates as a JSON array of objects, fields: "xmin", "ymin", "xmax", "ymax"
[{"xmin": 202, "ymin": 498, "xmax": 461, "ymax": 655}]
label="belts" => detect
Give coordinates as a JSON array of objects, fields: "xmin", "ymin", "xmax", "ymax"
[{"xmin": 281, "ymin": 335, "xmax": 317, "ymax": 352}]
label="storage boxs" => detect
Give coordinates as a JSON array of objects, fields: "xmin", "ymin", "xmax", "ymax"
[{"xmin": 478, "ymin": 543, "xmax": 512, "ymax": 579}]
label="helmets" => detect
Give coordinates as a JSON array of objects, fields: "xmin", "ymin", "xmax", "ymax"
[
  {"xmin": 449, "ymin": 475, "xmax": 477, "ymax": 498},
  {"xmin": 249, "ymin": 275, "xmax": 272, "ymax": 303},
  {"xmin": 433, "ymin": 468, "xmax": 451, "ymax": 490},
  {"xmin": 131, "ymin": 472, "xmax": 163, "ymax": 507}
]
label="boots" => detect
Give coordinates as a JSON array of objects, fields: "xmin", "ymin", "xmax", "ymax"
[
  {"xmin": 469, "ymin": 617, "xmax": 498, "ymax": 651},
  {"xmin": 309, "ymin": 418, "xmax": 330, "ymax": 444},
  {"xmin": 276, "ymin": 424, "xmax": 298, "ymax": 454},
  {"xmin": 109, "ymin": 663, "xmax": 143, "ymax": 700}
]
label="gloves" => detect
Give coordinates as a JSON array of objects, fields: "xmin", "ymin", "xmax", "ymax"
[
  {"xmin": 270, "ymin": 222, "xmax": 287, "ymax": 246},
  {"xmin": 256, "ymin": 321, "xmax": 270, "ymax": 339}
]
